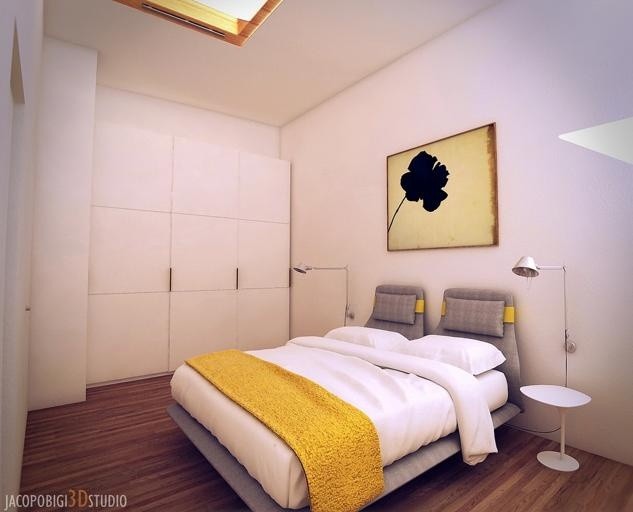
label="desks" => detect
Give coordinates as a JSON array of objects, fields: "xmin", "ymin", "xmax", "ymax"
[{"xmin": 517, "ymin": 385, "xmax": 592, "ymax": 474}]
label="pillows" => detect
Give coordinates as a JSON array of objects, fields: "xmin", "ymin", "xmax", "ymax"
[
  {"xmin": 324, "ymin": 325, "xmax": 409, "ymax": 356},
  {"xmin": 370, "ymin": 291, "xmax": 418, "ymax": 326},
  {"xmin": 401, "ymin": 333, "xmax": 506, "ymax": 378},
  {"xmin": 442, "ymin": 296, "xmax": 507, "ymax": 339}
]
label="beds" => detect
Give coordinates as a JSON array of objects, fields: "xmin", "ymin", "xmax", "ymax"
[{"xmin": 164, "ymin": 282, "xmax": 527, "ymax": 512}]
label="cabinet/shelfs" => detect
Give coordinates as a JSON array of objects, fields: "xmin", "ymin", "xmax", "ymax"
[
  {"xmin": 167, "ymin": 136, "xmax": 239, "ymax": 375},
  {"xmin": 236, "ymin": 148, "xmax": 293, "ymax": 351},
  {"xmin": 85, "ymin": 136, "xmax": 174, "ymax": 389}
]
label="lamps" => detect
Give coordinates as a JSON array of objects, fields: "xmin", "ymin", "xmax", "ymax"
[
  {"xmin": 293, "ymin": 262, "xmax": 353, "ymax": 329},
  {"xmin": 510, "ymin": 255, "xmax": 578, "ymax": 354}
]
